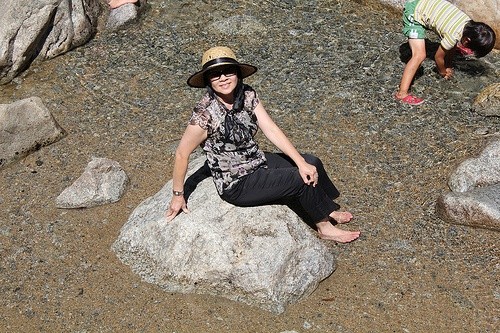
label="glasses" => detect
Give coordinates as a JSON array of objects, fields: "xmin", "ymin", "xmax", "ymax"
[{"xmin": 211, "ymin": 69, "xmax": 235, "ymax": 82}]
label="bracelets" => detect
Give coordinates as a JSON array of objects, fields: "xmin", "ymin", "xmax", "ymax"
[{"xmin": 173, "ymin": 189, "xmax": 185, "ymax": 195}]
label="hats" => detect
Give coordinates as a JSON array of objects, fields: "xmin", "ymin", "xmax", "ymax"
[{"xmin": 187, "ymin": 46, "xmax": 258, "ymax": 88}]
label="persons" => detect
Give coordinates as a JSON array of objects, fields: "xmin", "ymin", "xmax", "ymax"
[
  {"xmin": 166, "ymin": 45, "xmax": 362, "ymax": 244},
  {"xmin": 396, "ymin": 0, "xmax": 496, "ymax": 105}
]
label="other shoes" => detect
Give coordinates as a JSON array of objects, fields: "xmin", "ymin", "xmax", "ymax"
[
  {"xmin": 394, "ymin": 91, "xmax": 425, "ymax": 106},
  {"xmin": 455, "ymin": 42, "xmax": 472, "ymax": 57}
]
